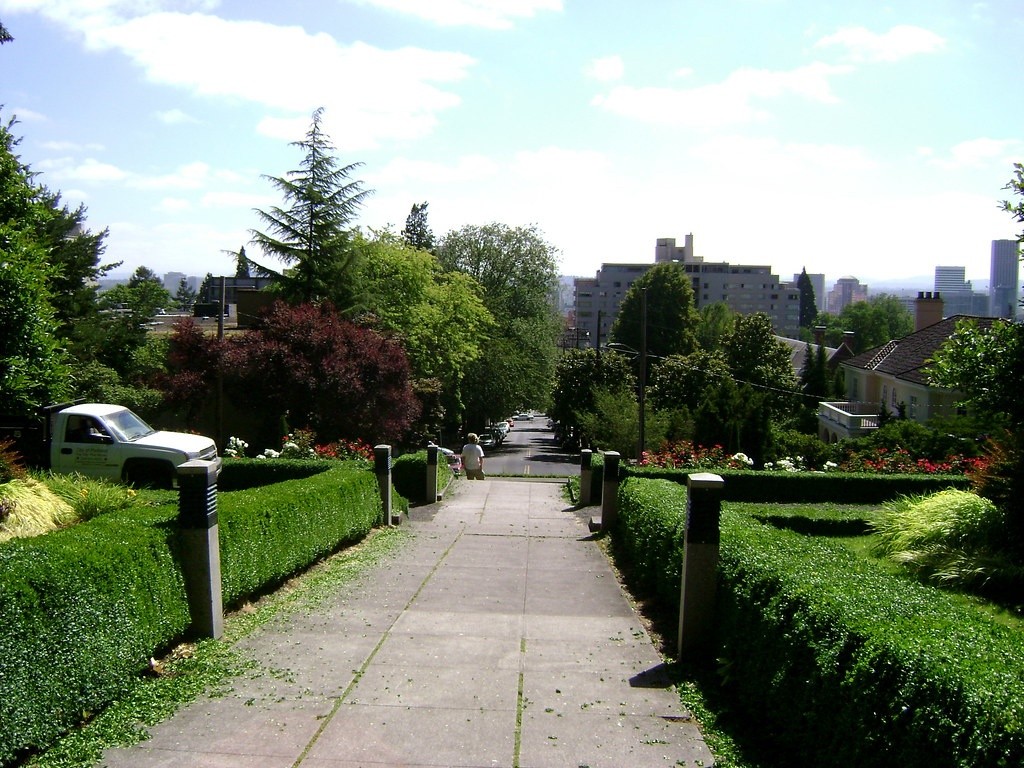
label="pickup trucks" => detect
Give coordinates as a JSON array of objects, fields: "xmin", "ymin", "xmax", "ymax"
[{"xmin": 0, "ymin": 398, "xmax": 222, "ymax": 490}]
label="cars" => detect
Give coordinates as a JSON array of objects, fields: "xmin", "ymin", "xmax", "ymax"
[
  {"xmin": 512, "ymin": 414, "xmax": 534, "ymax": 421},
  {"xmin": 446, "ymin": 455, "xmax": 463, "ymax": 473},
  {"xmin": 479, "ymin": 419, "xmax": 514, "ymax": 450}
]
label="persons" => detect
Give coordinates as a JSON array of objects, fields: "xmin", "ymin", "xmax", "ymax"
[
  {"xmin": 460, "ymin": 433, "xmax": 485, "ymax": 480},
  {"xmin": 85, "ymin": 418, "xmax": 112, "ymax": 443}
]
